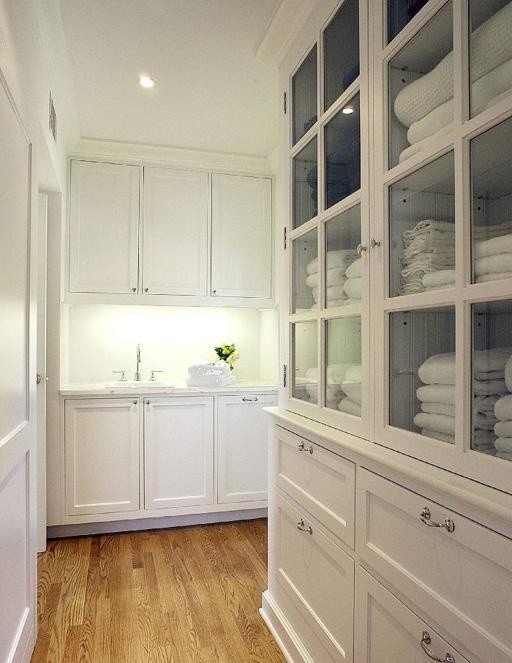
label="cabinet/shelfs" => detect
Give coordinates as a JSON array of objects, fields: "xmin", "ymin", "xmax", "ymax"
[
  {"xmin": 211, "ymin": 160, "xmax": 271, "ymax": 301},
  {"xmin": 261, "ymin": 408, "xmax": 511, "ymax": 662},
  {"xmin": 253, "ymin": 0, "xmax": 511, "ymax": 480},
  {"xmin": 63, "ymin": 151, "xmax": 210, "ymax": 301},
  {"xmin": 57, "ymin": 383, "xmax": 213, "ymax": 538},
  {"xmin": 213, "ymin": 381, "xmax": 279, "ymax": 529}
]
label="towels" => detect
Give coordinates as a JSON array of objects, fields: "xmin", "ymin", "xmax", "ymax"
[{"xmin": 304, "ymin": 0, "xmax": 510, "ymax": 459}]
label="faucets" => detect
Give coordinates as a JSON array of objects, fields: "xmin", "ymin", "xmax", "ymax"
[{"xmin": 134, "ymin": 344, "xmax": 143, "ymax": 382}]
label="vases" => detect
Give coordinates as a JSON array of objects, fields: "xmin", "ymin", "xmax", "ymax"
[{"xmin": 221, "ymin": 362, "xmax": 238, "ymax": 386}]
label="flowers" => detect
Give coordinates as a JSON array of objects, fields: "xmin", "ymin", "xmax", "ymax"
[{"xmin": 214, "ymin": 342, "xmax": 240, "ymax": 370}]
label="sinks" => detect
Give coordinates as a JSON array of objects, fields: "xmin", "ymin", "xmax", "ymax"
[{"xmin": 104, "ymin": 381, "xmax": 175, "ymax": 390}]
